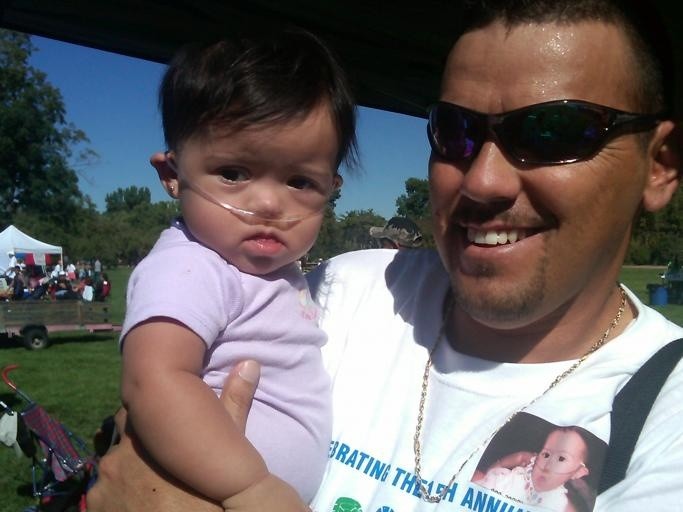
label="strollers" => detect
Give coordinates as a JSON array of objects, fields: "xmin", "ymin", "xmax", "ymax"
[{"xmin": 1, "ymin": 362, "xmax": 104, "ymax": 512}]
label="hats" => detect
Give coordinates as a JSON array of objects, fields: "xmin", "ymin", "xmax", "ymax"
[
  {"xmin": 7, "ymin": 251, "xmax": 15, "ymax": 255},
  {"xmin": 0, "ymin": 410, "xmax": 24, "ymax": 459}
]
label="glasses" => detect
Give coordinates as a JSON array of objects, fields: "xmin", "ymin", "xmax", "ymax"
[{"xmin": 424, "ymin": 97, "xmax": 659, "ymax": 170}]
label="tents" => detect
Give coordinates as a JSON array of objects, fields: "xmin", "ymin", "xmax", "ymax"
[{"xmin": 0, "ymin": 224, "xmax": 64, "ymax": 301}]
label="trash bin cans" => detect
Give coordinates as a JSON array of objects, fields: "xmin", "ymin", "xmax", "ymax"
[{"xmin": 648, "ymin": 284, "xmax": 669, "ymax": 305}]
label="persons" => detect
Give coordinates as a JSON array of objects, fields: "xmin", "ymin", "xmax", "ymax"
[
  {"xmin": 82, "ymin": 0, "xmax": 682, "ymax": 511},
  {"xmin": 118, "ymin": 17, "xmax": 365, "ymax": 512},
  {"xmin": 0, "ymin": 250, "xmax": 112, "ymax": 306},
  {"xmin": 470, "ymin": 425, "xmax": 589, "ymax": 511}
]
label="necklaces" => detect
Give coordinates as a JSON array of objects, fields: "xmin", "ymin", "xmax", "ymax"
[{"xmin": 411, "ymin": 282, "xmax": 625, "ymax": 501}]
